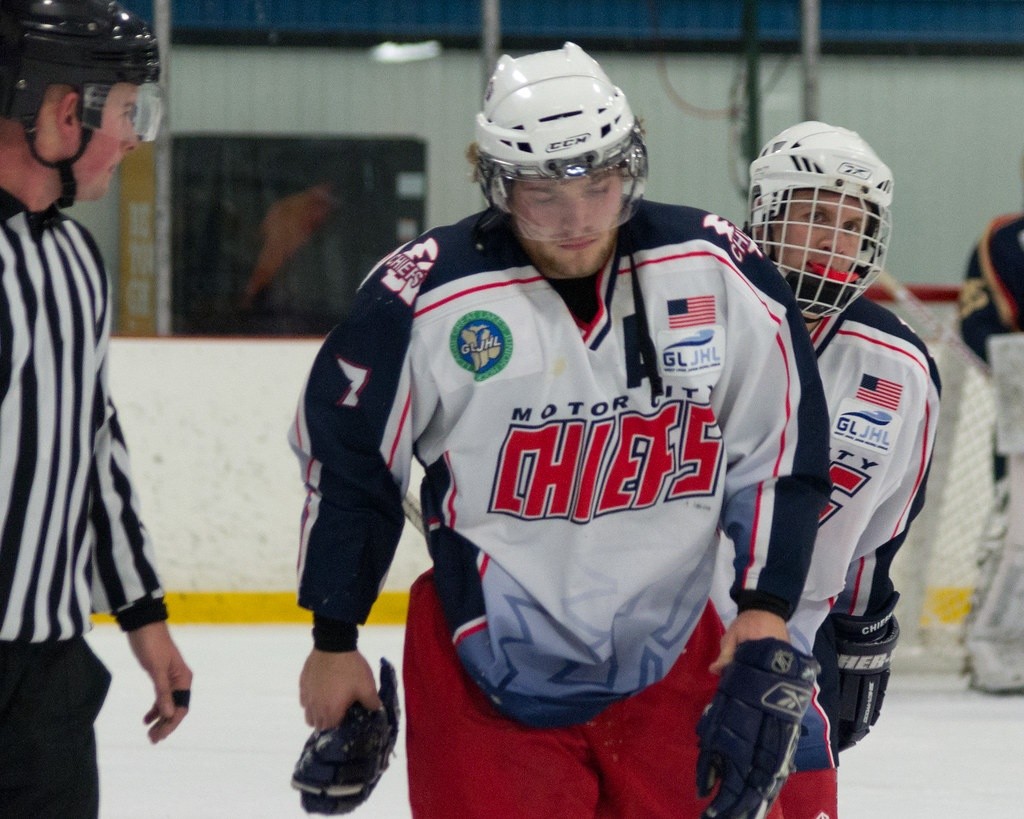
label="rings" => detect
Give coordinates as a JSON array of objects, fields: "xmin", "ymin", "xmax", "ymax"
[{"xmin": 171, "ymin": 688, "xmax": 190, "ymax": 710}]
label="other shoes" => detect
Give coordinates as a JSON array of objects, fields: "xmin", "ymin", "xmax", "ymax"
[{"xmin": 961, "ymin": 632, "xmax": 1023, "ymax": 693}]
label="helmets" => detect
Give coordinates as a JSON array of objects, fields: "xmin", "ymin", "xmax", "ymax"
[
  {"xmin": 474, "ymin": 41, "xmax": 637, "ymax": 180},
  {"xmin": 2, "ymin": 0, "xmax": 160, "ymax": 119},
  {"xmin": 747, "ymin": 121, "xmax": 895, "ymax": 216}
]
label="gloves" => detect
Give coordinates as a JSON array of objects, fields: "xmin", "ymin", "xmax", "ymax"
[
  {"xmin": 292, "ymin": 656, "xmax": 399, "ymax": 815},
  {"xmin": 829, "ymin": 588, "xmax": 902, "ymax": 751},
  {"xmin": 695, "ymin": 637, "xmax": 819, "ymax": 819}
]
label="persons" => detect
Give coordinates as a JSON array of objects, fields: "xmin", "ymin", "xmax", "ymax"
[
  {"xmin": 288, "ymin": 38, "xmax": 831, "ymax": 818},
  {"xmin": 955, "ymin": 212, "xmax": 1024, "ymax": 694},
  {"xmin": 1, "ymin": 0, "xmax": 193, "ymax": 817},
  {"xmin": 708, "ymin": 122, "xmax": 944, "ymax": 818}
]
